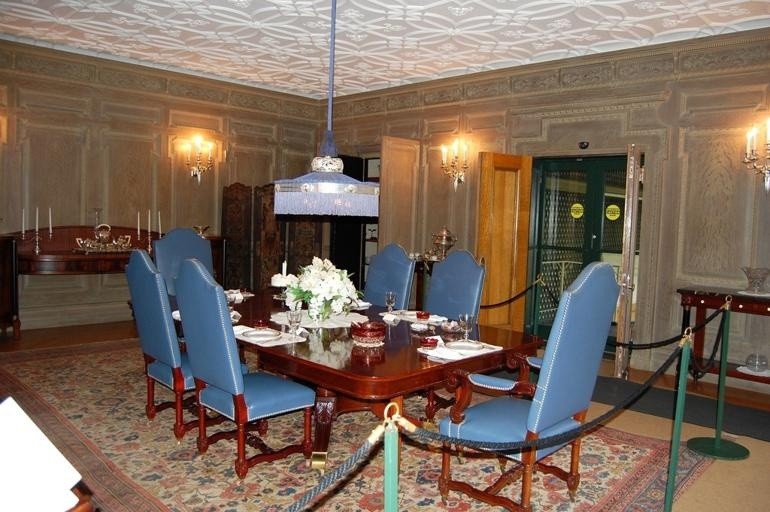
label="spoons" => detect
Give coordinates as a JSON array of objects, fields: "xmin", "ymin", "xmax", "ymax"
[{"xmin": 430, "ymin": 326, "xmax": 437, "ymax": 335}]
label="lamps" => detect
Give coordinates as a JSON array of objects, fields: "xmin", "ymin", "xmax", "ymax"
[
  {"xmin": 175, "ymin": 133, "xmax": 216, "ymax": 186},
  {"xmin": 743, "ymin": 117, "xmax": 770, "ymax": 195},
  {"xmin": 440, "ymin": 140, "xmax": 470, "ymax": 192}
]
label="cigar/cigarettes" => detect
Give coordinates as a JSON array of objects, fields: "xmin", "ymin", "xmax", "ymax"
[{"xmin": 351, "ymin": 317, "xmax": 361, "ymax": 330}]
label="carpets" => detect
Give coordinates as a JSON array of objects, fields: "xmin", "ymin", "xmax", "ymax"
[{"xmin": 0, "ymin": 338, "xmax": 719, "ymax": 512}]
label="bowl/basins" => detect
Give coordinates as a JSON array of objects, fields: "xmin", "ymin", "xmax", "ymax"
[
  {"xmin": 421, "ymin": 336, "xmax": 438, "ymax": 351},
  {"xmin": 442, "ymin": 321, "xmax": 462, "ymax": 333},
  {"xmin": 349, "ymin": 345, "xmax": 386, "ymax": 367},
  {"xmin": 412, "ymin": 323, "xmax": 428, "ymax": 333},
  {"xmin": 350, "ymin": 320, "xmax": 386, "ymax": 344}
]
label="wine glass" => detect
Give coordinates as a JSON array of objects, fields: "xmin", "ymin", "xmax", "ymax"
[
  {"xmin": 385, "ymin": 292, "xmax": 396, "ymax": 314},
  {"xmin": 286, "ymin": 311, "xmax": 302, "ymax": 339},
  {"xmin": 288, "ymin": 337, "xmax": 298, "ymax": 358},
  {"xmin": 459, "ymin": 313, "xmax": 475, "ymax": 344}
]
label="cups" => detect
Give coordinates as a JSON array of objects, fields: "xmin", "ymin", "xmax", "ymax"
[
  {"xmin": 240, "ymin": 288, "xmax": 249, "ymax": 295},
  {"xmin": 409, "ymin": 252, "xmax": 438, "ymax": 261},
  {"xmin": 416, "ymin": 311, "xmax": 430, "ymax": 320}
]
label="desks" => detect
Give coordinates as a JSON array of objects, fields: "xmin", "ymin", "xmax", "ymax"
[{"xmin": 677, "ymin": 285, "xmax": 770, "ymax": 386}]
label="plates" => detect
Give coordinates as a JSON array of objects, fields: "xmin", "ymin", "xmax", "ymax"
[
  {"xmin": 242, "ymin": 329, "xmax": 283, "ymax": 341},
  {"xmin": 352, "ymin": 302, "xmax": 372, "ymax": 310},
  {"xmin": 446, "ymin": 341, "xmax": 484, "ymax": 351}
]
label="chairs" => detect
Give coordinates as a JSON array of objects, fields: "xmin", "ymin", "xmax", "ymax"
[
  {"xmin": 364, "ymin": 243, "xmax": 416, "ymax": 309},
  {"xmin": 152, "ymin": 227, "xmax": 215, "ymax": 309},
  {"xmin": 426, "ymin": 250, "xmax": 484, "ymax": 334},
  {"xmin": 172, "ymin": 258, "xmax": 314, "ymax": 479},
  {"xmin": 439, "ymin": 257, "xmax": 619, "ymax": 512},
  {"xmin": 123, "ymin": 248, "xmax": 234, "ymax": 438}
]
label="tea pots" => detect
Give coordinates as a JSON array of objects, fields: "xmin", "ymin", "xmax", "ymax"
[{"xmin": 91, "ymin": 224, "xmax": 113, "ymax": 244}]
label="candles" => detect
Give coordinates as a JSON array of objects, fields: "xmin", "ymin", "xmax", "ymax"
[
  {"xmin": 136, "ymin": 207, "xmax": 163, "ymax": 253},
  {"xmin": 22, "ymin": 206, "xmax": 54, "ymax": 254}
]
